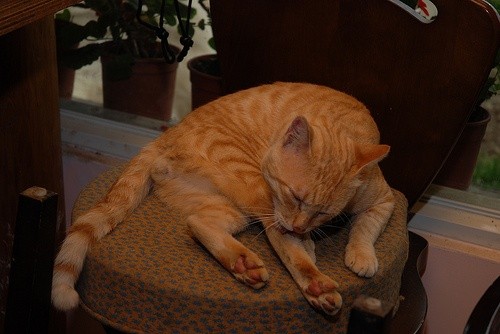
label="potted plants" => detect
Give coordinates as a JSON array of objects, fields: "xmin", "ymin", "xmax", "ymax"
[
  {"xmin": 54, "ymin": 0, "xmax": 221, "ymax": 122},
  {"xmin": 436, "ymin": 0, "xmax": 500, "ymax": 192}
]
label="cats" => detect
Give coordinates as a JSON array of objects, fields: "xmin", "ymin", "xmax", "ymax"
[{"xmin": 50, "ymin": 80, "xmax": 397, "ymax": 316}]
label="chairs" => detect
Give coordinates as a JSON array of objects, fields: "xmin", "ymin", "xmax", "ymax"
[{"xmin": 71, "ymin": 0, "xmax": 500, "ymax": 334}]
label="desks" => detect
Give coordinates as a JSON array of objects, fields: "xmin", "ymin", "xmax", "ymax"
[{"xmin": 0, "ymin": 0, "xmax": 85, "ymax": 334}]
